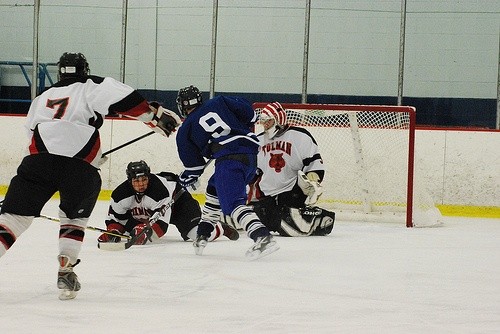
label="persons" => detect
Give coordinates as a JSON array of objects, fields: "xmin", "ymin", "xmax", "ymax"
[
  {"xmin": 0, "ymin": 53, "xmax": 184, "ymax": 300},
  {"xmin": 98, "ymin": 84, "xmax": 336, "ymax": 260}
]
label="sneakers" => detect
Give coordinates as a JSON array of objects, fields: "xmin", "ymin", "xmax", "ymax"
[
  {"xmin": 57, "ymin": 255, "xmax": 81, "ymax": 300},
  {"xmin": 218, "ymin": 220, "xmax": 239, "ymax": 241},
  {"xmin": 245, "ymin": 233, "xmax": 280, "ymax": 260},
  {"xmin": 193, "ymin": 235, "xmax": 208, "ymax": 256}
]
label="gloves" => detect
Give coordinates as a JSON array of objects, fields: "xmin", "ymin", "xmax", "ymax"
[
  {"xmin": 97, "ymin": 229, "xmax": 121, "ymax": 243},
  {"xmin": 127, "ymin": 224, "xmax": 153, "ymax": 245},
  {"xmin": 98, "ymin": 154, "xmax": 108, "ymax": 166},
  {"xmin": 143, "ymin": 101, "xmax": 182, "ymax": 139},
  {"xmin": 186, "ymin": 181, "xmax": 201, "ymax": 194}
]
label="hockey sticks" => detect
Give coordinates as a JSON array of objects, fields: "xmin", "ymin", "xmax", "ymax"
[
  {"xmin": 97, "ymin": 157, "xmax": 215, "ymax": 251},
  {"xmin": 102, "ymin": 121, "xmax": 174, "ymax": 157},
  {"xmin": 0, "ymin": 202, "xmax": 132, "ymax": 239}
]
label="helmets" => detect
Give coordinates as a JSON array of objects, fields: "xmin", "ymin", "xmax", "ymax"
[
  {"xmin": 58, "ymin": 52, "xmax": 90, "ymax": 77},
  {"xmin": 254, "ymin": 102, "xmax": 287, "ymax": 147},
  {"xmin": 126, "ymin": 160, "xmax": 151, "ymax": 196},
  {"xmin": 176, "ymin": 85, "xmax": 204, "ymax": 119}
]
